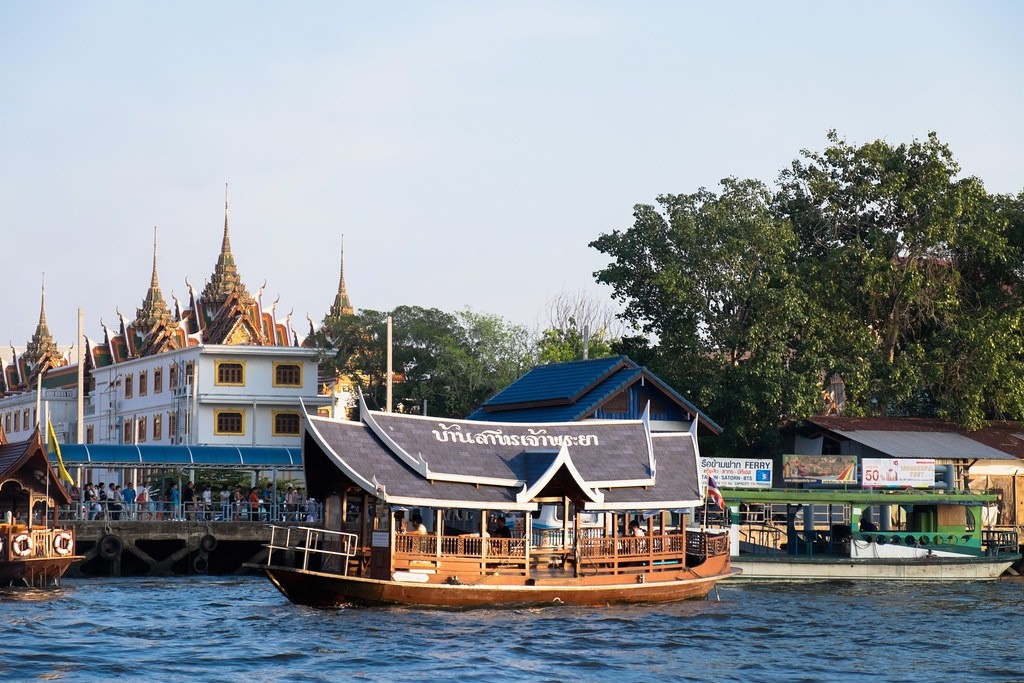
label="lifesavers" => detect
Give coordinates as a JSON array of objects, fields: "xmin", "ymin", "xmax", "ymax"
[
  {"xmin": 54, "ymin": 533, "xmax": 74, "ymax": 556},
  {"xmin": 12, "ymin": 534, "xmax": 33, "ymax": 556},
  {"xmin": 201, "ymin": 534, "xmax": 217, "ymax": 552},
  {"xmin": 97, "ymin": 535, "xmax": 124, "ymax": 559},
  {"xmin": 194, "ymin": 556, "xmax": 209, "ymax": 574}
]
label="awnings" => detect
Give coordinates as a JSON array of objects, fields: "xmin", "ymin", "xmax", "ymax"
[{"xmin": 806, "ymin": 417, "xmax": 1024, "ymax": 460}]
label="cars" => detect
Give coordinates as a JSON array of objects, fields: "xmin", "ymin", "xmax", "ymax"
[
  {"xmin": 695, "ymin": 503, "xmax": 732, "ymax": 524},
  {"xmin": 772, "ymin": 514, "xmax": 804, "ymax": 525},
  {"xmin": 738, "ymin": 502, "xmax": 766, "ymax": 525}
]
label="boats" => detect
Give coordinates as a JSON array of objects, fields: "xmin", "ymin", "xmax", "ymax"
[
  {"xmin": 242, "ymin": 386, "xmax": 743, "ymax": 610},
  {"xmin": 0, "ymin": 421, "xmax": 86, "ymax": 582},
  {"xmin": 530, "ymin": 490, "xmax": 1022, "ymax": 584}
]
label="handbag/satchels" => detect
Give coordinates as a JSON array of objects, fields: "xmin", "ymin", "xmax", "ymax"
[{"xmin": 137, "ymin": 493, "xmax": 146, "ymax": 502}]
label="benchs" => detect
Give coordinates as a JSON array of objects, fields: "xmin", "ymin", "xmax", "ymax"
[{"xmin": 336, "ymin": 528, "xmax": 732, "ymax": 575}]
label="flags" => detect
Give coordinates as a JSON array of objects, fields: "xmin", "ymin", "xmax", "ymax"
[
  {"xmin": 47, "ymin": 420, "xmax": 74, "ymax": 485},
  {"xmin": 707, "ymin": 474, "xmax": 725, "ymax": 509}
]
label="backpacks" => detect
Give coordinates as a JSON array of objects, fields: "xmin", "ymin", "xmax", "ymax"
[{"xmin": 228, "ymin": 490, "xmax": 238, "ymax": 506}]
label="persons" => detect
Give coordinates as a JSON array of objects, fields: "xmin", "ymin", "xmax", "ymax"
[
  {"xmin": 406, "ymin": 514, "xmax": 427, "ymax": 548},
  {"xmin": 219, "ymin": 485, "xmax": 231, "ymax": 520},
  {"xmin": 165, "ymin": 482, "xmax": 180, "ymax": 520},
  {"xmin": 83, "ymin": 481, "xmax": 107, "ymax": 520},
  {"xmin": 69, "ymin": 481, "xmax": 79, "ymax": 518},
  {"xmin": 860, "ymin": 518, "xmax": 877, "ymax": 542},
  {"xmin": 494, "ymin": 517, "xmax": 511, "ymax": 552},
  {"xmin": 183, "ymin": 481, "xmax": 197, "ymax": 521},
  {"xmin": 233, "ymin": 485, "xmax": 245, "ymax": 522},
  {"xmin": 35, "ymin": 511, "xmax": 62, "ymax": 520},
  {"xmin": 249, "ymin": 487, "xmax": 260, "ymax": 520},
  {"xmin": 202, "ymin": 485, "xmax": 212, "ymax": 521},
  {"xmin": 277, "ymin": 487, "xmax": 284, "ymax": 521},
  {"xmin": 628, "ymin": 520, "xmax": 644, "ymax": 552},
  {"xmin": 459, "ymin": 522, "xmax": 490, "ymax": 553},
  {"xmin": 262, "ymin": 484, "xmax": 273, "ymax": 521},
  {"xmin": 285, "ymin": 486, "xmax": 306, "ymax": 521},
  {"xmin": 108, "ymin": 481, "xmax": 164, "ymax": 521}
]
